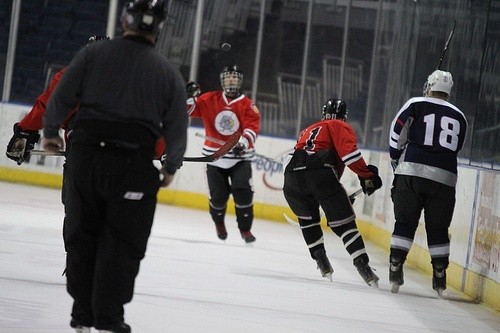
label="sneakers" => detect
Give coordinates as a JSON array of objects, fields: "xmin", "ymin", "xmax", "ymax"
[
  {"xmin": 241, "ymin": 230, "xmax": 256, "ymax": 245},
  {"xmin": 314, "ymin": 249, "xmax": 334, "ymax": 281},
  {"xmin": 389, "ymin": 257, "xmax": 405, "ymax": 293},
  {"xmin": 216, "ymin": 222, "xmax": 227, "ymax": 242},
  {"xmin": 355, "ymin": 258, "xmax": 378, "ymax": 289},
  {"xmin": 432, "ymin": 264, "xmax": 446, "ymax": 296}
]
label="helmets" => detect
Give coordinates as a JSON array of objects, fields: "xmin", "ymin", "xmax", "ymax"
[
  {"xmin": 121, "ymin": 0, "xmax": 168, "ymax": 33},
  {"xmin": 322, "ymin": 99, "xmax": 347, "ymax": 122},
  {"xmin": 423, "ymin": 70, "xmax": 453, "ymax": 101},
  {"xmin": 220, "ymin": 64, "xmax": 244, "ymax": 96}
]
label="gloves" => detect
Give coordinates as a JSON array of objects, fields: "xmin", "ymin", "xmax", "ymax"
[
  {"xmin": 186, "ymin": 80, "xmax": 201, "ymax": 100},
  {"xmin": 6, "ymin": 122, "xmax": 41, "ymax": 165},
  {"xmin": 231, "ymin": 144, "xmax": 248, "ymax": 156},
  {"xmin": 358, "ymin": 165, "xmax": 382, "ymax": 195}
]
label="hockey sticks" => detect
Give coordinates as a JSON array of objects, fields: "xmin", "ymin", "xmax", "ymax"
[
  {"xmin": 282, "ymin": 181, "xmax": 371, "ymax": 227},
  {"xmin": 190, "ymin": 131, "xmax": 292, "ymax": 164},
  {"xmin": 29, "ymin": 126, "xmax": 244, "ymax": 163}
]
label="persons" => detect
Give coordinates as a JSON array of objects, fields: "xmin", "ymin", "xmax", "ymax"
[
  {"xmin": 185, "ymin": 64, "xmax": 262, "ymax": 242},
  {"xmin": 6, "ymin": 0, "xmax": 188, "ymax": 333},
  {"xmin": 283, "ymin": 99, "xmax": 383, "ymax": 288},
  {"xmin": 389, "ymin": 70, "xmax": 468, "ymax": 296}
]
